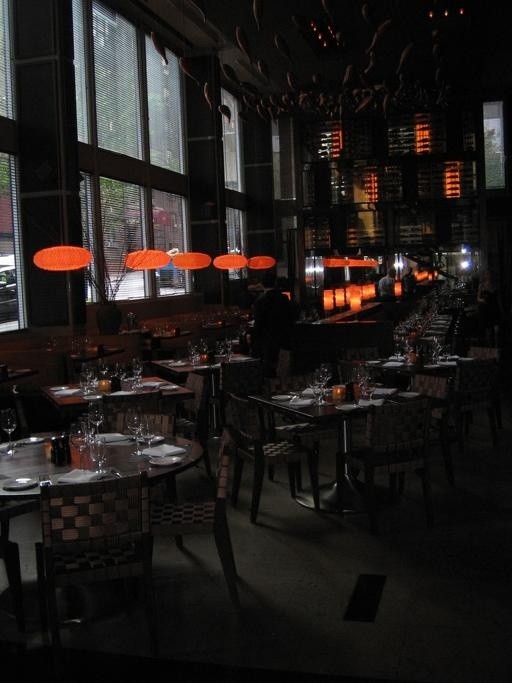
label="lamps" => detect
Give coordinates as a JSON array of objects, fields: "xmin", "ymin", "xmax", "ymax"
[{"xmin": 34, "ymin": 245, "xmax": 402, "ymax": 311}]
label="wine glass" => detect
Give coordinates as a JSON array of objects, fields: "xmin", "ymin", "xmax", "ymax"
[
  {"xmin": 124, "ymin": 406, "xmax": 156, "ymax": 452},
  {"xmin": 69, "ymin": 399, "xmax": 109, "ymax": 475},
  {"xmin": 363, "ymin": 375, "xmax": 376, "ymax": 401},
  {"xmin": 0, "ymin": 407, "xmax": 18, "ymax": 443},
  {"xmin": 186, "ymin": 339, "xmax": 200, "ymax": 365},
  {"xmin": 214, "ymin": 336, "xmax": 233, "ymax": 362},
  {"xmin": 79, "ymin": 357, "xmax": 144, "ymax": 394},
  {"xmin": 305, "ymin": 361, "xmax": 334, "ymax": 407}
]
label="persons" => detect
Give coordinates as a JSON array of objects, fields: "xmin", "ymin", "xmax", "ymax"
[
  {"xmin": 378, "ymin": 267, "xmax": 397, "ymax": 296},
  {"xmin": 401, "ymin": 266, "xmax": 417, "ymax": 295},
  {"xmin": 232, "ymin": 278, "xmax": 296, "ymax": 377}
]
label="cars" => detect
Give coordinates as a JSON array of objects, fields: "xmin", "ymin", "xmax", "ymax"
[{"xmin": 0, "ymin": 266, "xmax": 19, "ymax": 324}]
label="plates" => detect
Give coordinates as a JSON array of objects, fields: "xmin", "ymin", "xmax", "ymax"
[
  {"xmin": 82, "ymin": 395, "xmax": 101, "ymax": 399},
  {"xmin": 48, "ymin": 385, "xmax": 70, "ymax": 390},
  {"xmin": 158, "ymin": 384, "xmax": 180, "ymax": 391},
  {"xmin": 398, "ymin": 391, "xmax": 421, "ymax": 398},
  {"xmin": 335, "ymin": 402, "xmax": 361, "ymax": 411},
  {"xmin": 0, "ymin": 475, "xmax": 38, "ymax": 491},
  {"xmin": 271, "ymin": 394, "xmax": 292, "ymax": 401},
  {"xmin": 147, "ymin": 454, "xmax": 183, "ymax": 465},
  {"xmin": 16, "ymin": 436, "xmax": 44, "ymax": 444},
  {"xmin": 136, "ymin": 434, "xmax": 165, "ymax": 444}
]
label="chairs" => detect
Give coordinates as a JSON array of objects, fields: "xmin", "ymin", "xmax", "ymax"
[
  {"xmin": 5, "ymin": 340, "xmax": 262, "ymax": 648},
  {"xmin": 221, "ymin": 338, "xmax": 501, "ymax": 524}
]
